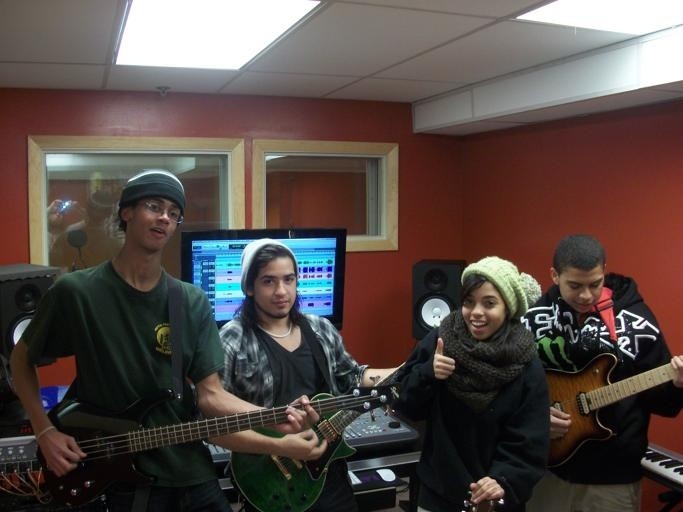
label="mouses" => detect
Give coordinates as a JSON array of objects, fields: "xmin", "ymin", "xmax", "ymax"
[{"xmin": 376, "ymin": 469, "xmax": 395, "ymax": 482}]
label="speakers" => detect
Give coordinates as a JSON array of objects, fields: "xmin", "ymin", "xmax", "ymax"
[
  {"xmin": 1, "ymin": 263, "xmax": 71, "ymax": 421},
  {"xmin": 412, "ymin": 259, "xmax": 468, "ymax": 341}
]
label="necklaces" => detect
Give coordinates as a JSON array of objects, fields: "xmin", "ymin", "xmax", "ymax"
[{"xmin": 256, "ymin": 322, "xmax": 293, "ymax": 338}]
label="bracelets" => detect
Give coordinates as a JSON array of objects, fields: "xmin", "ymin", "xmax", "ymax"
[{"xmin": 32, "ymin": 424, "xmax": 60, "ymax": 446}]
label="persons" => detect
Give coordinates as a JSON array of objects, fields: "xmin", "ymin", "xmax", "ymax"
[
  {"xmin": 516, "ymin": 233, "xmax": 683, "ymax": 512},
  {"xmin": 11, "ymin": 167, "xmax": 323, "ymax": 511},
  {"xmin": 49, "ymin": 179, "xmax": 193, "ymax": 280},
  {"xmin": 391, "ymin": 256, "xmax": 550, "ymax": 511},
  {"xmin": 199, "ymin": 235, "xmax": 409, "ymax": 512}
]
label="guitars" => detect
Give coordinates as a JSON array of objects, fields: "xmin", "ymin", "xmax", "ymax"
[
  {"xmin": 226, "ymin": 363, "xmax": 407, "ymax": 512},
  {"xmin": 35, "ymin": 381, "xmax": 403, "ymax": 512},
  {"xmin": 545, "ymin": 354, "xmax": 679, "ymax": 468}
]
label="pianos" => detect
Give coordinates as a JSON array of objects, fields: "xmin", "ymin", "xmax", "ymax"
[{"xmin": 640, "ymin": 442, "xmax": 682, "ymax": 492}]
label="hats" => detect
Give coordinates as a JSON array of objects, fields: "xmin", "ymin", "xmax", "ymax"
[
  {"xmin": 462, "ymin": 255, "xmax": 542, "ymax": 321},
  {"xmin": 120, "ymin": 168, "xmax": 185, "ymax": 214},
  {"xmin": 239, "ymin": 239, "xmax": 299, "ymax": 295}
]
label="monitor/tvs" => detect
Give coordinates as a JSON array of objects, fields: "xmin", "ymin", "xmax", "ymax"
[{"xmin": 179, "ymin": 227, "xmax": 347, "ymax": 329}]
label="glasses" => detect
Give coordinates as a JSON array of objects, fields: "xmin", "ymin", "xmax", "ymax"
[{"xmin": 136, "ymin": 202, "xmax": 183, "ymax": 227}]
